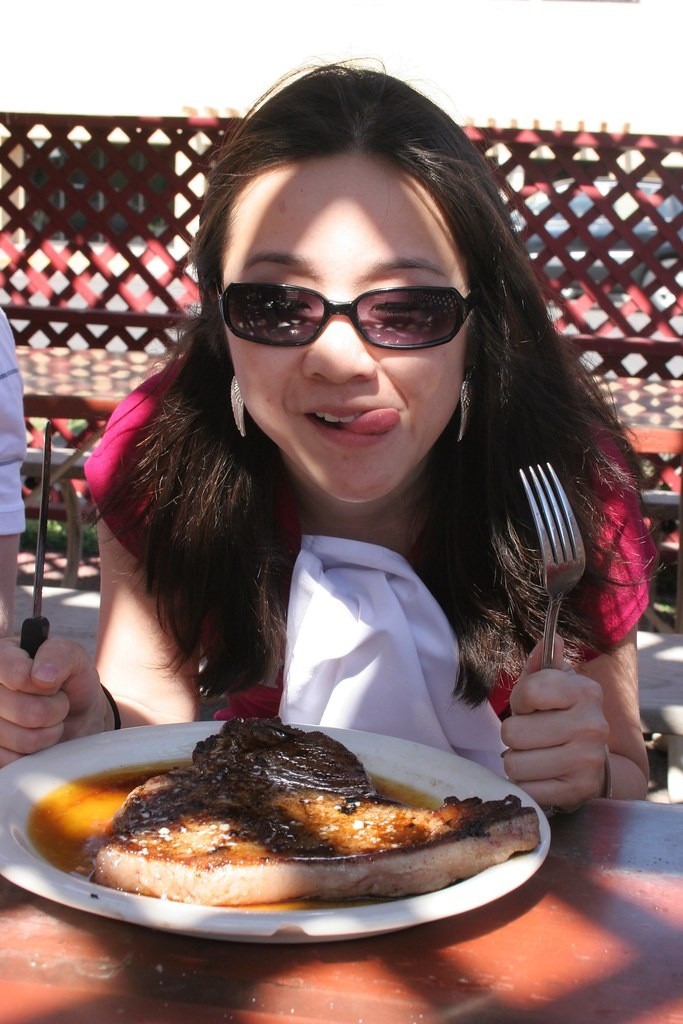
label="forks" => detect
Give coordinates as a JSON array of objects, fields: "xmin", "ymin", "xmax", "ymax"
[{"xmin": 519, "ymin": 463, "xmax": 585, "ymax": 668}]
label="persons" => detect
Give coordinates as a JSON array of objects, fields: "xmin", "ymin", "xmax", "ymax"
[
  {"xmin": 0, "ymin": 56, "xmax": 658, "ymax": 806},
  {"xmin": 0, "ymin": 304, "xmax": 27, "ymax": 639}
]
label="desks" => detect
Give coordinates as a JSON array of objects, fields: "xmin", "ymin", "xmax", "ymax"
[{"xmin": 0, "ymin": 794, "xmax": 683, "ymax": 1024}]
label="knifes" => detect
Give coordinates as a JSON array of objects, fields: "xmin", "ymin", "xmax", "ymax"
[{"xmin": 20, "ymin": 421, "xmax": 51, "ymax": 659}]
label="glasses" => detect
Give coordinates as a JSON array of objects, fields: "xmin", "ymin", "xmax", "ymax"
[{"xmin": 215, "ymin": 270, "xmax": 481, "ymax": 351}]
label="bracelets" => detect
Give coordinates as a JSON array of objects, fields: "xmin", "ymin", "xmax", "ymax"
[
  {"xmin": 101, "ymin": 684, "xmax": 121, "ymax": 730},
  {"xmin": 605, "ymin": 747, "xmax": 613, "ymax": 799}
]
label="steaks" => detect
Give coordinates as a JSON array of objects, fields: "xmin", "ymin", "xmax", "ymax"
[
  {"xmin": 90, "ymin": 763, "xmax": 541, "ymax": 910},
  {"xmin": 192, "ymin": 712, "xmax": 377, "ymax": 802}
]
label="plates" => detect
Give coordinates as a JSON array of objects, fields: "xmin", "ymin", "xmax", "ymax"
[{"xmin": 0, "ymin": 718, "xmax": 552, "ymax": 945}]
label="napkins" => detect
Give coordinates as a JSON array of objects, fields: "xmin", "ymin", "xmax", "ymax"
[{"xmin": 280, "ymin": 536, "xmax": 510, "ymax": 780}]
